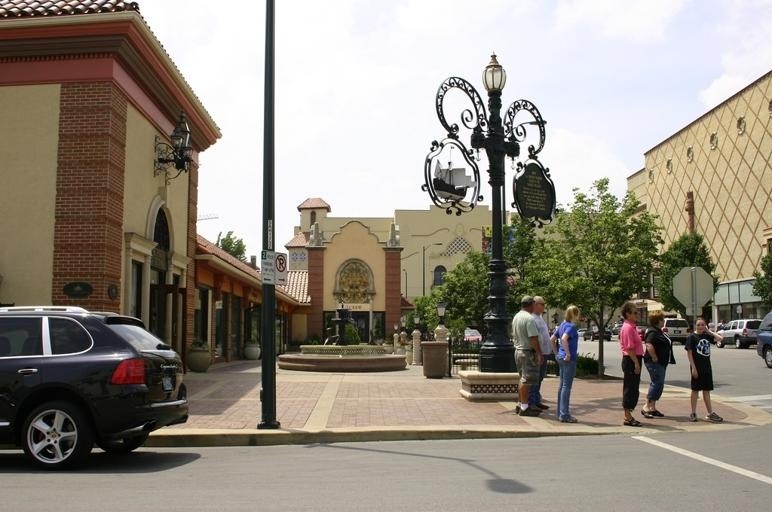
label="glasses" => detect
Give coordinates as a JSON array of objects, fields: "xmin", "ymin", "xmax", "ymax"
[
  {"xmin": 630, "ymin": 311, "xmax": 638, "ymax": 315},
  {"xmin": 538, "ymin": 303, "xmax": 545, "ymax": 306}
]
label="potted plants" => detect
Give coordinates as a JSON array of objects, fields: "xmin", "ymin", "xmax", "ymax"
[
  {"xmin": 186, "ymin": 337, "xmax": 214, "ymax": 373},
  {"xmin": 243, "ymin": 339, "xmax": 262, "ymax": 360}
]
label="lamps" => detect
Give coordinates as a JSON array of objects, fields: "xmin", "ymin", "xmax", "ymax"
[
  {"xmin": 150, "ymin": 108, "xmax": 195, "ymax": 186},
  {"xmin": 434, "ymin": 301, "xmax": 447, "ymax": 325},
  {"xmin": 412, "ymin": 315, "xmax": 421, "ymax": 329},
  {"xmin": 400, "ymin": 320, "xmax": 406, "ymax": 331},
  {"xmin": 392, "ymin": 320, "xmax": 400, "ymax": 333}
]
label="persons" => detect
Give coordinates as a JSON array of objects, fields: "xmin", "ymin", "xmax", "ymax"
[
  {"xmin": 549, "ymin": 303, "xmax": 581, "ymax": 423},
  {"xmin": 720, "ymin": 319, "xmax": 726, "ymax": 329},
  {"xmin": 510, "ymin": 296, "xmax": 544, "ymax": 416},
  {"xmin": 685, "ymin": 316, "xmax": 724, "ymax": 422},
  {"xmin": 529, "ymin": 295, "xmax": 551, "ymax": 412},
  {"xmin": 641, "ymin": 310, "xmax": 675, "ymax": 418},
  {"xmin": 618, "ymin": 302, "xmax": 644, "ymax": 426}
]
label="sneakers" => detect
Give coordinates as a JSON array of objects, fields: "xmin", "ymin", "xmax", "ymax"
[
  {"xmin": 689, "ymin": 413, "xmax": 697, "ymax": 422},
  {"xmin": 641, "ymin": 409, "xmax": 664, "ymax": 418},
  {"xmin": 515, "ymin": 403, "xmax": 550, "ymax": 416},
  {"xmin": 705, "ymin": 411, "xmax": 723, "ymax": 422},
  {"xmin": 556, "ymin": 414, "xmax": 578, "ymax": 423}
]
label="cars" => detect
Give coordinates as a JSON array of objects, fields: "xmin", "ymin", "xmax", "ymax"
[{"xmin": 577, "ymin": 323, "xmax": 648, "ymax": 341}]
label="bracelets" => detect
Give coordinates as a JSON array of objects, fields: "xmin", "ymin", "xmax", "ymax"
[{"xmin": 709, "ymin": 331, "xmax": 714, "ymax": 336}]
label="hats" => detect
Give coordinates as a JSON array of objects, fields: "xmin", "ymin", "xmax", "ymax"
[{"xmin": 521, "ymin": 295, "xmax": 536, "ymax": 304}]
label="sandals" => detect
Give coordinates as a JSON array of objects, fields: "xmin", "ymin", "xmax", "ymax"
[{"xmin": 624, "ymin": 417, "xmax": 642, "ymax": 426}]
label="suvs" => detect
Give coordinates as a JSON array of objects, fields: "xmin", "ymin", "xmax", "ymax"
[
  {"xmin": 756, "ymin": 310, "xmax": 772, "ymax": 368},
  {"xmin": 0, "ymin": 302, "xmax": 190, "ymax": 472},
  {"xmin": 715, "ymin": 318, "xmax": 763, "ymax": 349},
  {"xmin": 662, "ymin": 318, "xmax": 691, "ymax": 345}
]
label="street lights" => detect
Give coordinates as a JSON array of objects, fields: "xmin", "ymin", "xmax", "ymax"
[
  {"xmin": 422, "ymin": 241, "xmax": 443, "ymax": 297},
  {"xmin": 402, "ymin": 268, "xmax": 407, "ymax": 298},
  {"xmin": 434, "ymin": 300, "xmax": 449, "ymax": 342},
  {"xmin": 393, "ymin": 315, "xmax": 423, "ymax": 366},
  {"xmin": 460, "ymin": 54, "xmax": 521, "ymax": 401}
]
